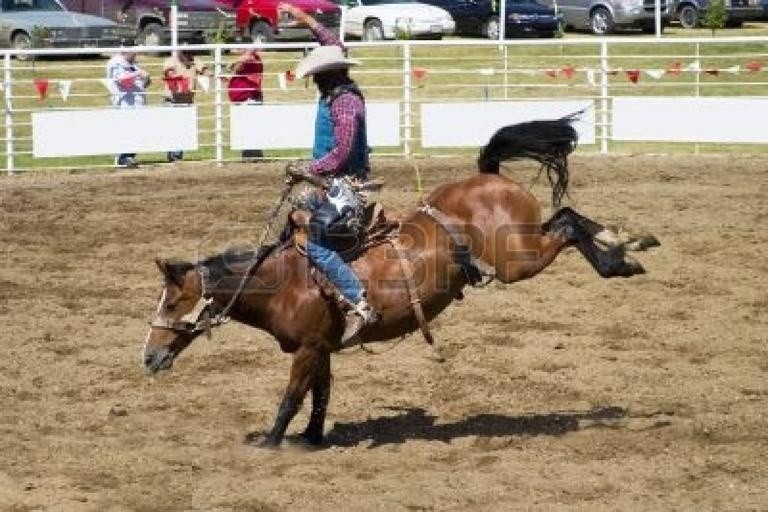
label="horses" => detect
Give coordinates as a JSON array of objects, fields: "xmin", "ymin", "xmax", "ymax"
[{"xmin": 142, "ymin": 105, "xmax": 662, "ymax": 449}]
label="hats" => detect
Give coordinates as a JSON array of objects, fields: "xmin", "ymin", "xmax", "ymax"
[{"xmin": 296, "ymin": 45, "xmax": 362, "ymax": 80}]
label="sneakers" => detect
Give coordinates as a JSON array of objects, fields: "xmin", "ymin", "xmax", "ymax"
[{"xmin": 342, "ymin": 301, "xmax": 374, "ymax": 344}]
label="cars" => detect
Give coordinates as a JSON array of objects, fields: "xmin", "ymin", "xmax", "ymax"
[
  {"xmin": 425, "ymin": 0, "xmax": 566, "ymax": 45},
  {"xmin": 336, "ymin": 1, "xmax": 458, "ymax": 43},
  {"xmin": 666, "ymin": 0, "xmax": 767, "ymax": 31},
  {"xmin": 221, "ymin": 1, "xmax": 346, "ymax": 50},
  {"xmin": 0, "ymin": 0, "xmax": 125, "ymax": 62},
  {"xmin": 541, "ymin": 0, "xmax": 676, "ymax": 37},
  {"xmin": 61, "ymin": 0, "xmax": 239, "ymax": 60}
]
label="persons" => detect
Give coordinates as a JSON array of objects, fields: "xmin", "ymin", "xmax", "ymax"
[
  {"xmin": 107, "ymin": 39, "xmax": 151, "ymax": 165},
  {"xmin": 277, "ymin": 1, "xmax": 378, "ymax": 346},
  {"xmin": 228, "ymin": 35, "xmax": 264, "ymax": 163},
  {"xmin": 162, "ymin": 41, "xmax": 215, "ymax": 162}
]
